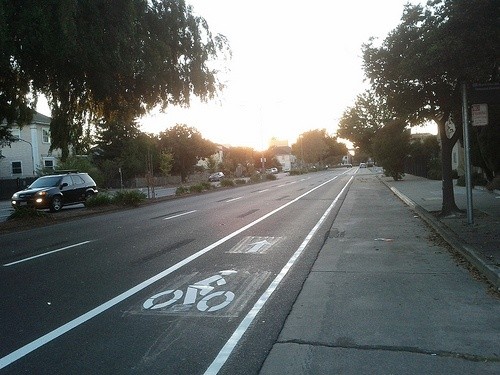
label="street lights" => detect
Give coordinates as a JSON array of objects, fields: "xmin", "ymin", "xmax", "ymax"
[
  {"xmin": 14, "ymin": 138, "xmax": 35, "ymax": 177},
  {"xmin": 299, "ymin": 134, "xmax": 304, "ymax": 160}
]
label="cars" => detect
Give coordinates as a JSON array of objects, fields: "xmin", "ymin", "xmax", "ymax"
[
  {"xmin": 347, "ymin": 164, "xmax": 352, "ymax": 168},
  {"xmin": 256, "ymin": 168, "xmax": 279, "ymax": 175},
  {"xmin": 284, "ymin": 167, "xmax": 290, "ymax": 172},
  {"xmin": 360, "ymin": 163, "xmax": 366, "ymax": 168},
  {"xmin": 209, "ymin": 172, "xmax": 225, "ymax": 182}
]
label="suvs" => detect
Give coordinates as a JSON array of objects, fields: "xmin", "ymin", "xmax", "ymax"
[{"xmin": 11, "ymin": 171, "xmax": 98, "ymax": 213}]
